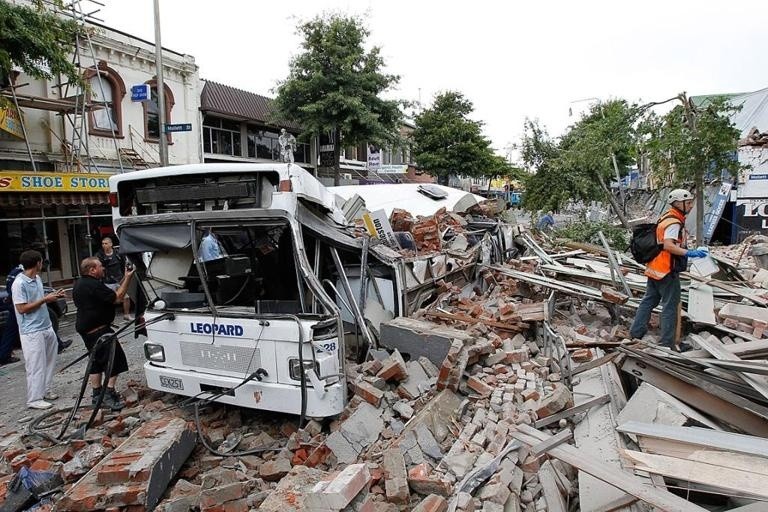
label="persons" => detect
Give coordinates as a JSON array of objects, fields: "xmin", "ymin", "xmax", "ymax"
[
  {"xmin": 627, "ymin": 187, "xmax": 710, "ymax": 353},
  {"xmin": 11, "ymin": 249, "xmax": 66, "ymax": 411},
  {"xmin": 94, "ymin": 236, "xmax": 137, "ymax": 324},
  {"xmin": 72, "ymin": 256, "xmax": 137, "ymax": 410},
  {"xmin": 0, "ymin": 263, "xmax": 74, "ymax": 365},
  {"xmin": 199, "ymin": 230, "xmax": 224, "ymax": 262},
  {"xmin": 537, "ymin": 211, "xmax": 554, "ymax": 230}
]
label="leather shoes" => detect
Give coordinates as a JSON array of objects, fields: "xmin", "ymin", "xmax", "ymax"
[
  {"xmin": 59, "ymin": 340, "xmax": 72, "ymax": 354},
  {"xmin": 679, "ymin": 342, "xmax": 694, "ymax": 354}
]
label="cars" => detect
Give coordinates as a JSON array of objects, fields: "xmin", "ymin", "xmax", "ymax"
[{"xmin": 0, "ymin": 286, "xmax": 67, "ymax": 335}]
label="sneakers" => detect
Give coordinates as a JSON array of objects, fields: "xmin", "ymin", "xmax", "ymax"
[
  {"xmin": 92, "ymin": 391, "xmax": 127, "ymax": 411},
  {"xmin": 27, "ymin": 389, "xmax": 58, "ymax": 410},
  {"xmin": 123, "ymin": 314, "xmax": 134, "ymax": 322}
]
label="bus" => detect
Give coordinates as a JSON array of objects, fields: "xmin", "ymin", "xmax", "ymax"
[{"xmin": 107, "ymin": 162, "xmax": 512, "ymax": 422}]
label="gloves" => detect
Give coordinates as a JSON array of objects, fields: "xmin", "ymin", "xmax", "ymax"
[{"xmin": 685, "ymin": 248, "xmax": 708, "ymax": 258}]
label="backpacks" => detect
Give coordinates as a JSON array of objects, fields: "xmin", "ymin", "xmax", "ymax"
[{"xmin": 630, "ymin": 211, "xmax": 684, "ymax": 264}]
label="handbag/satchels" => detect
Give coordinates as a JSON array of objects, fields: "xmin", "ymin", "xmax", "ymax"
[{"xmin": 671, "ymin": 247, "xmax": 687, "ymax": 272}]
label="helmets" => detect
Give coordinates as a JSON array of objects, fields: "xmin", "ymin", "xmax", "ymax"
[{"xmin": 666, "ymin": 188, "xmax": 694, "ymax": 204}]
